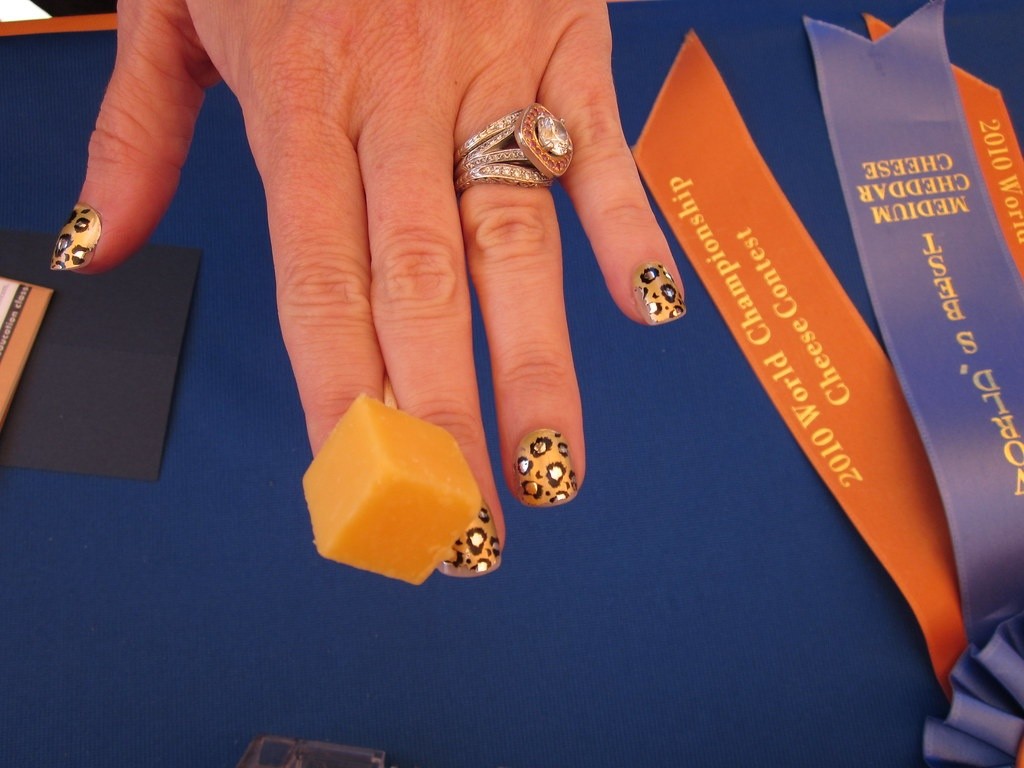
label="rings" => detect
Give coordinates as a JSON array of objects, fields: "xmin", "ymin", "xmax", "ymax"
[{"xmin": 454, "ymin": 102, "xmax": 573, "ymax": 190}]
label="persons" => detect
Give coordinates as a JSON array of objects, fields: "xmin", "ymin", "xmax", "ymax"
[{"xmin": 46, "ymin": 0, "xmax": 685, "ymax": 579}]
